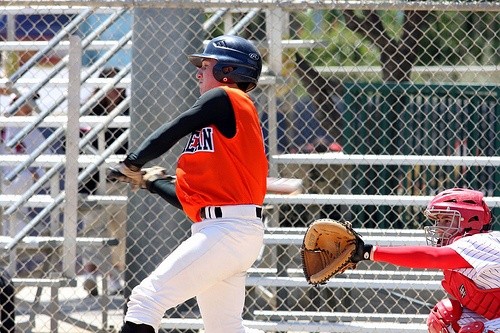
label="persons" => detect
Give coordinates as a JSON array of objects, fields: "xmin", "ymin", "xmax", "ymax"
[
  {"xmin": 62, "ymin": 65, "xmax": 130, "ymax": 192},
  {"xmin": 350, "ymin": 186, "xmax": 500, "ymax": 332},
  {"xmin": 0, "ymin": 87, "xmax": 56, "ymax": 236},
  {"xmin": 105, "ymin": 34, "xmax": 269, "ymax": 332}
]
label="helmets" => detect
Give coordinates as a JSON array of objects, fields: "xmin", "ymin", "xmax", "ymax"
[
  {"xmin": 428, "ymin": 188, "xmax": 491, "ymax": 240},
  {"xmin": 187, "ymin": 34, "xmax": 263, "ymax": 94}
]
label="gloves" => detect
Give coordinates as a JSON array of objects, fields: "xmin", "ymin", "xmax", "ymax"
[
  {"xmin": 138, "ymin": 166, "xmax": 171, "ymax": 189},
  {"xmin": 107, "ymin": 163, "xmax": 145, "ymax": 186}
]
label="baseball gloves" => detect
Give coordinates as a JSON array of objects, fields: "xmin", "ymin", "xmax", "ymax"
[{"xmin": 302, "ymin": 218, "xmax": 373, "ymax": 285}]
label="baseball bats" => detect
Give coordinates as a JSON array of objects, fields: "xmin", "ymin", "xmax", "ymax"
[{"xmin": 107, "ymin": 167, "xmax": 303, "ymax": 194}]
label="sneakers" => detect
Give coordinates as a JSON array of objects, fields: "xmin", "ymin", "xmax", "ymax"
[
  {"xmin": 108, "ymin": 280, "xmax": 124, "ymax": 295},
  {"xmin": 84, "ymin": 278, "xmax": 98, "ymax": 297}
]
